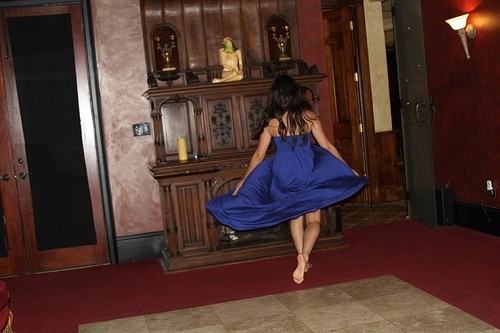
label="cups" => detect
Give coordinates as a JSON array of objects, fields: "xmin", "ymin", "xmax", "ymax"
[{"xmin": 177, "ymin": 137, "xmax": 188, "ymax": 161}]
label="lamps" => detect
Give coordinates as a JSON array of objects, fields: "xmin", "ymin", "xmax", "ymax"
[{"xmin": 445, "ymin": 13, "xmax": 476, "ymax": 59}]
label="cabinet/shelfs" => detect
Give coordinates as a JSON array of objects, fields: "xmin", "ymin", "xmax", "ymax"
[{"xmin": 141, "ymin": 73, "xmax": 347, "ymax": 271}]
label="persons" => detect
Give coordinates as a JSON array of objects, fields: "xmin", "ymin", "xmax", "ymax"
[
  {"xmin": 206, "ymin": 74, "xmax": 365, "ymax": 284},
  {"xmin": 212, "ymin": 38, "xmax": 243, "ymax": 83}
]
